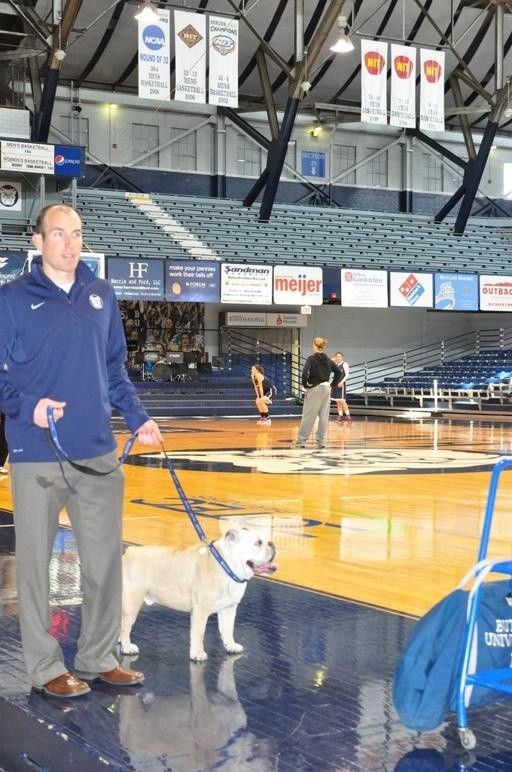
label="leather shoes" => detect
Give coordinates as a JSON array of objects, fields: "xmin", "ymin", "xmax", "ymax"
[
  {"xmin": 74, "ymin": 662, "xmax": 145, "ymax": 686},
  {"xmin": 32, "ymin": 673, "xmax": 91, "ymax": 698}
]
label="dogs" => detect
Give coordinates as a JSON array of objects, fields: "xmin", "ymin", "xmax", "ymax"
[{"xmin": 116, "ymin": 526, "xmax": 276, "ymax": 660}]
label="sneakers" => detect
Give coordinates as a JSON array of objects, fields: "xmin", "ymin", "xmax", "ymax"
[
  {"xmin": 337, "ymin": 415, "xmax": 351, "ymax": 421},
  {"xmin": 255, "ymin": 417, "xmax": 271, "ymax": 424},
  {"xmin": 290, "ymin": 442, "xmax": 325, "ymax": 448}
]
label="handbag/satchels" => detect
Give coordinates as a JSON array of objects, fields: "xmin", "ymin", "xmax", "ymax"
[{"xmin": 389, "ymin": 555, "xmax": 512, "ymax": 733}]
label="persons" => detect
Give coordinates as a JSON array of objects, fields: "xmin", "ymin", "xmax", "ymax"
[
  {"xmin": 1, "ymin": 203, "xmax": 169, "ymax": 699},
  {"xmin": 328, "ymin": 352, "xmax": 351, "ymax": 422},
  {"xmin": 117, "ymin": 299, "xmax": 206, "ymax": 371},
  {"xmin": 289, "ymin": 336, "xmax": 343, "ymax": 449},
  {"xmin": 250, "ymin": 364, "xmax": 278, "ymax": 425}
]
